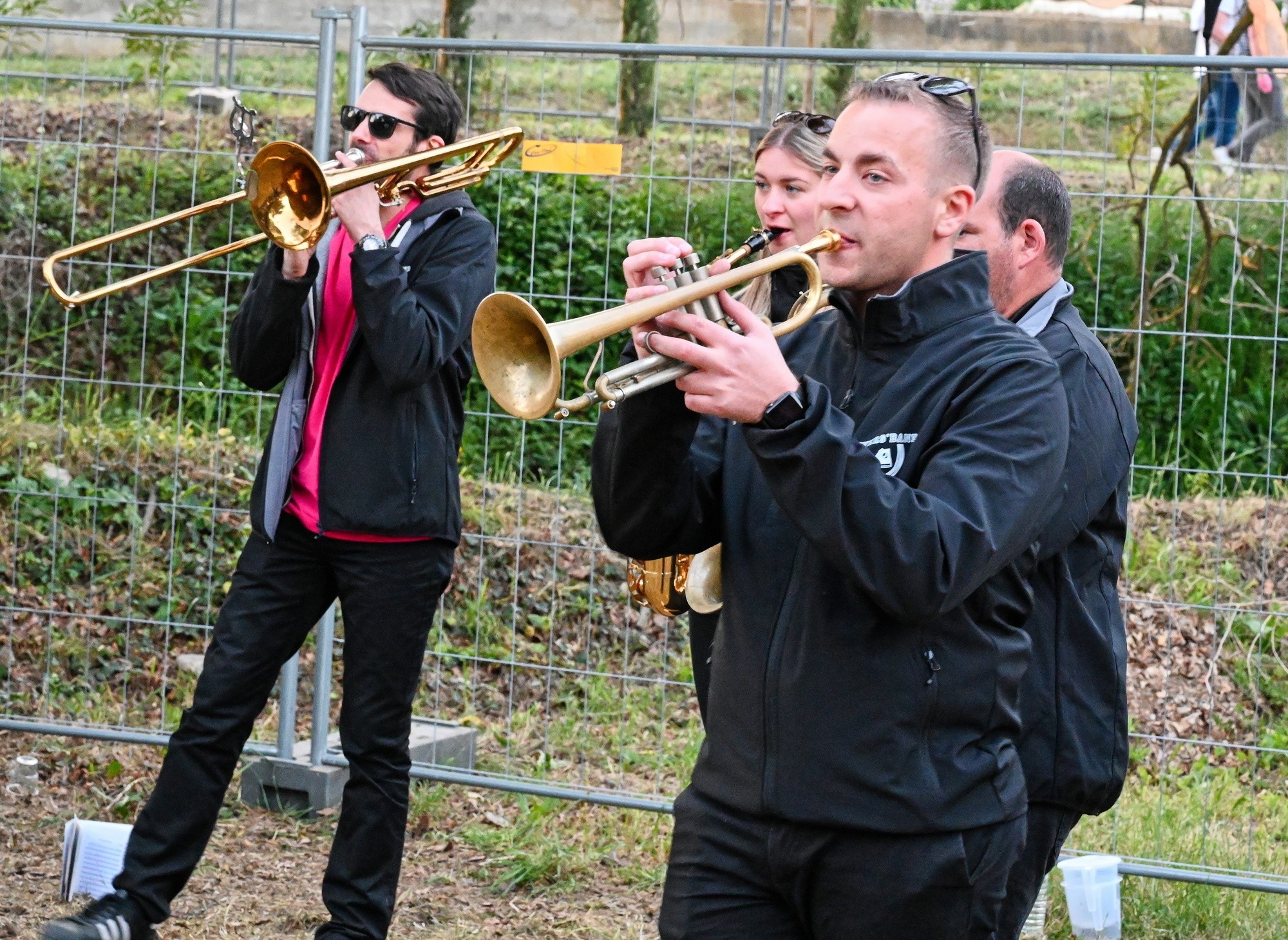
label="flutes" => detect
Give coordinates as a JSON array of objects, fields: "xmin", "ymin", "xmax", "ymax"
[{"xmin": 707, "ymin": 225, "xmax": 784, "ymax": 267}]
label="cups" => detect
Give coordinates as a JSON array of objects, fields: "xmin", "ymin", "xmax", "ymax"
[
  {"xmin": 1056, "ymin": 855, "xmax": 1123, "ymax": 940},
  {"xmin": 7, "ymin": 754, "xmax": 39, "ymax": 798}
]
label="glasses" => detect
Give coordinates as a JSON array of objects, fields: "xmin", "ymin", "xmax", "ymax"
[
  {"xmin": 772, "ymin": 111, "xmax": 836, "ymax": 134},
  {"xmin": 874, "ymin": 71, "xmax": 982, "ymax": 191},
  {"xmin": 340, "ymin": 105, "xmax": 426, "ymax": 139}
]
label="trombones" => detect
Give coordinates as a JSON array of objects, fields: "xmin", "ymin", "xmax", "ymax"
[{"xmin": 39, "ymin": 123, "xmax": 528, "ymax": 312}]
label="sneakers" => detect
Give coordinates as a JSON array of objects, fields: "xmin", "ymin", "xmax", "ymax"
[{"xmin": 39, "ymin": 890, "xmax": 159, "ymax": 940}]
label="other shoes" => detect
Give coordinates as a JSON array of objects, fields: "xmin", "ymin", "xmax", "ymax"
[
  {"xmin": 1212, "ymin": 146, "xmax": 1236, "ymax": 179},
  {"xmin": 1150, "ymin": 147, "xmax": 1172, "ymax": 174}
]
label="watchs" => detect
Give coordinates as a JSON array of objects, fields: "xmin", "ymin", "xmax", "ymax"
[{"xmin": 354, "ymin": 233, "xmax": 388, "ymax": 252}]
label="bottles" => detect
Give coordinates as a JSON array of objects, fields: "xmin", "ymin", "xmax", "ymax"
[{"xmin": 1020, "ymin": 873, "xmax": 1049, "ymax": 936}]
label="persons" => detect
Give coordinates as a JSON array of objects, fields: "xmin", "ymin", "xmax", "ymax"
[
  {"xmin": 41, "ymin": 61, "xmax": 496, "ymax": 939},
  {"xmin": 591, "ymin": 0, "xmax": 1287, "ymax": 940}
]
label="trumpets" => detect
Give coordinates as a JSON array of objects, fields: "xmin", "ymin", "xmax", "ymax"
[{"xmin": 467, "ymin": 225, "xmax": 844, "ymax": 422}]
